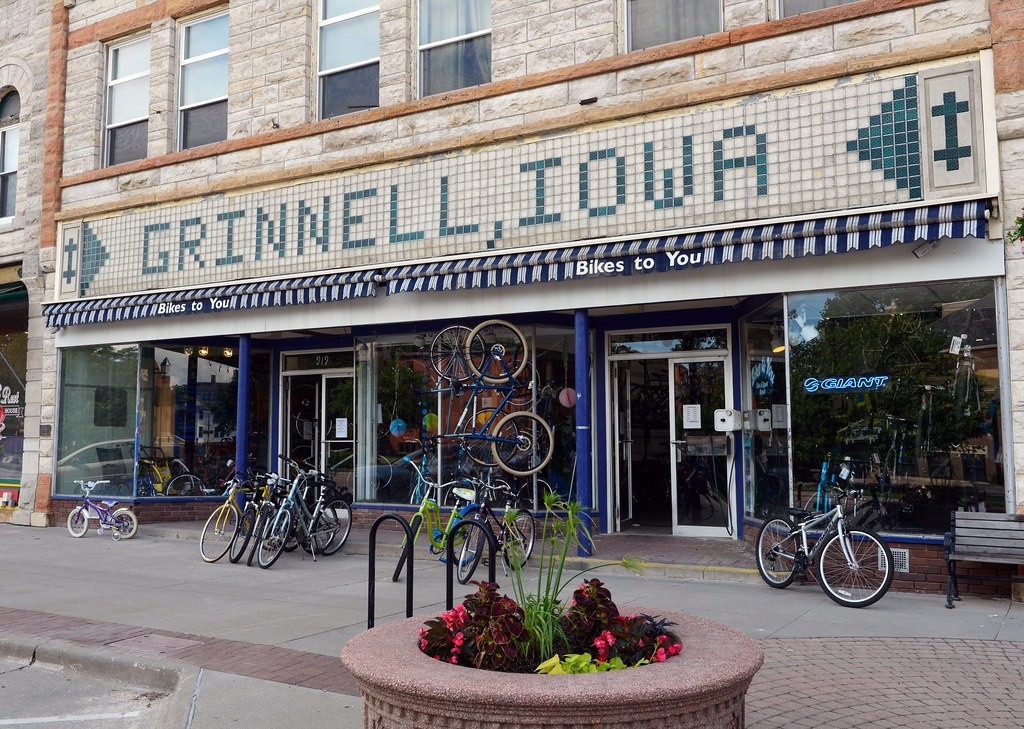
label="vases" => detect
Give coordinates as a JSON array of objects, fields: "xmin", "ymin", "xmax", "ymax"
[{"xmin": 340, "ymin": 605, "xmax": 766, "ymax": 729}]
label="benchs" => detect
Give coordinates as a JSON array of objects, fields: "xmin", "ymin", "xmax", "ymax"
[{"xmin": 944, "ymin": 510, "xmax": 1024, "ymax": 610}]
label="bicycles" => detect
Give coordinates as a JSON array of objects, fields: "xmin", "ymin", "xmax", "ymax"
[
  {"xmin": 197, "ymin": 384, "xmax": 392, "ymax": 569},
  {"xmin": 754, "ymin": 484, "xmax": 894, "ymax": 609},
  {"xmin": 163, "ymin": 461, "xmax": 236, "ymax": 496},
  {"xmin": 393, "ymin": 319, "xmax": 556, "ymax": 585},
  {"xmin": 66, "ymin": 479, "xmax": 138, "ymax": 542}
]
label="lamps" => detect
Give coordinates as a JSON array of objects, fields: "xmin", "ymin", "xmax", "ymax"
[
  {"xmin": 421, "ymin": 393, "xmax": 441, "ymax": 433},
  {"xmin": 389, "ymin": 347, "xmax": 407, "ymax": 438},
  {"xmin": 769, "ymin": 308, "xmax": 819, "ymax": 353},
  {"xmin": 413, "ymin": 333, "xmax": 428, "ymax": 347},
  {"xmin": 559, "ymin": 336, "xmax": 578, "ymax": 409},
  {"xmin": 451, "ymin": 334, "xmax": 462, "ymax": 346},
  {"xmin": 482, "ymin": 326, "xmax": 498, "ymax": 346},
  {"xmin": 358, "ymin": 344, "xmax": 371, "ymax": 362},
  {"xmin": 183, "ymin": 346, "xmax": 234, "ymax": 358}
]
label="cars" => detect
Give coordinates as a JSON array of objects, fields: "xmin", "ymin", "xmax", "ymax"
[
  {"xmin": 354, "ymin": 441, "xmax": 547, "ymax": 509},
  {"xmin": 54, "ymin": 437, "xmax": 169, "ymax": 496},
  {"xmin": 134, "ymin": 433, "xmax": 205, "ymax": 464}
]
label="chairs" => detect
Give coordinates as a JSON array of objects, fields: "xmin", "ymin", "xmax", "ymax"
[{"xmin": 94, "ymin": 448, "xmax": 134, "ymax": 496}]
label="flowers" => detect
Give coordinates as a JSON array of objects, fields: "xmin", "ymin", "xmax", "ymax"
[{"xmin": 419, "ymin": 575, "xmax": 684, "ymax": 670}]
